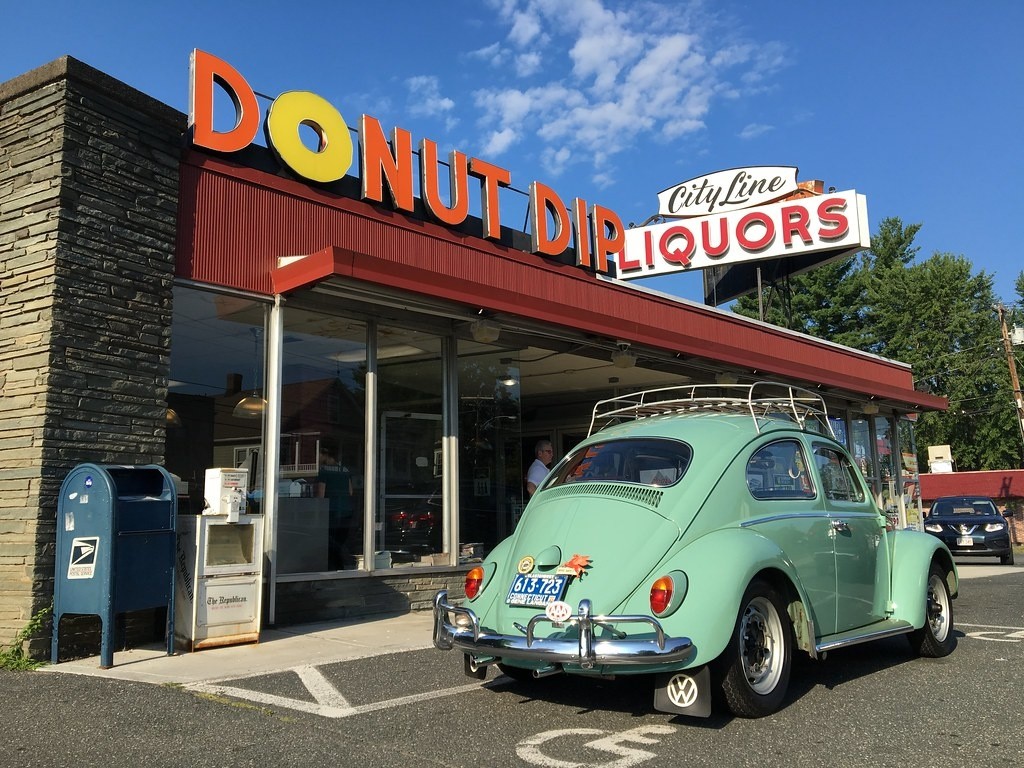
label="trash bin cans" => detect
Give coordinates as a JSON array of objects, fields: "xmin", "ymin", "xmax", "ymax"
[{"xmin": 51, "ymin": 463, "xmax": 179, "ymax": 669}]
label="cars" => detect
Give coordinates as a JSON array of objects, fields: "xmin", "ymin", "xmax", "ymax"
[
  {"xmin": 431, "ymin": 379, "xmax": 960, "ymax": 719},
  {"xmin": 385, "ymin": 505, "xmax": 447, "ymax": 554},
  {"xmin": 922, "ymin": 495, "xmax": 1015, "ymax": 566}
]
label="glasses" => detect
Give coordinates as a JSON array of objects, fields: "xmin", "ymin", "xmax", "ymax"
[{"xmin": 543, "ymin": 450, "xmax": 553, "ymax": 455}]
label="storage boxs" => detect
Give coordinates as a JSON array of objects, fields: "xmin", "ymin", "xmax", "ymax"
[
  {"xmin": 175, "ymin": 513, "xmax": 267, "ymax": 655},
  {"xmin": 203, "ymin": 467, "xmax": 249, "ymax": 517}
]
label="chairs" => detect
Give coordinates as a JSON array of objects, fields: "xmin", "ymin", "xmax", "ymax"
[{"xmin": 939, "ymin": 504, "xmax": 954, "ymax": 515}]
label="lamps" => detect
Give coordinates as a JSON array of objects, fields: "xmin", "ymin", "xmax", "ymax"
[
  {"xmin": 471, "ymin": 317, "xmax": 503, "ymax": 344},
  {"xmin": 716, "ymin": 371, "xmax": 738, "ymax": 384},
  {"xmin": 232, "ymin": 326, "xmax": 266, "ymax": 421},
  {"xmin": 497, "ymin": 374, "xmax": 522, "ymax": 386},
  {"xmin": 611, "ymin": 349, "xmax": 638, "ymax": 369},
  {"xmin": 167, "ymin": 408, "xmax": 183, "ymax": 429},
  {"xmin": 862, "ymin": 401, "xmax": 881, "ymax": 415},
  {"xmin": 796, "ymin": 390, "xmax": 816, "ymax": 404}
]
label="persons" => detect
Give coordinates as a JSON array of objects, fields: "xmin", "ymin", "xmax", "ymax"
[
  {"xmin": 974, "ymin": 505, "xmax": 986, "ymax": 515},
  {"xmin": 316, "ymin": 441, "xmax": 355, "ymax": 571},
  {"xmin": 527, "ymin": 439, "xmax": 553, "ymax": 498}
]
